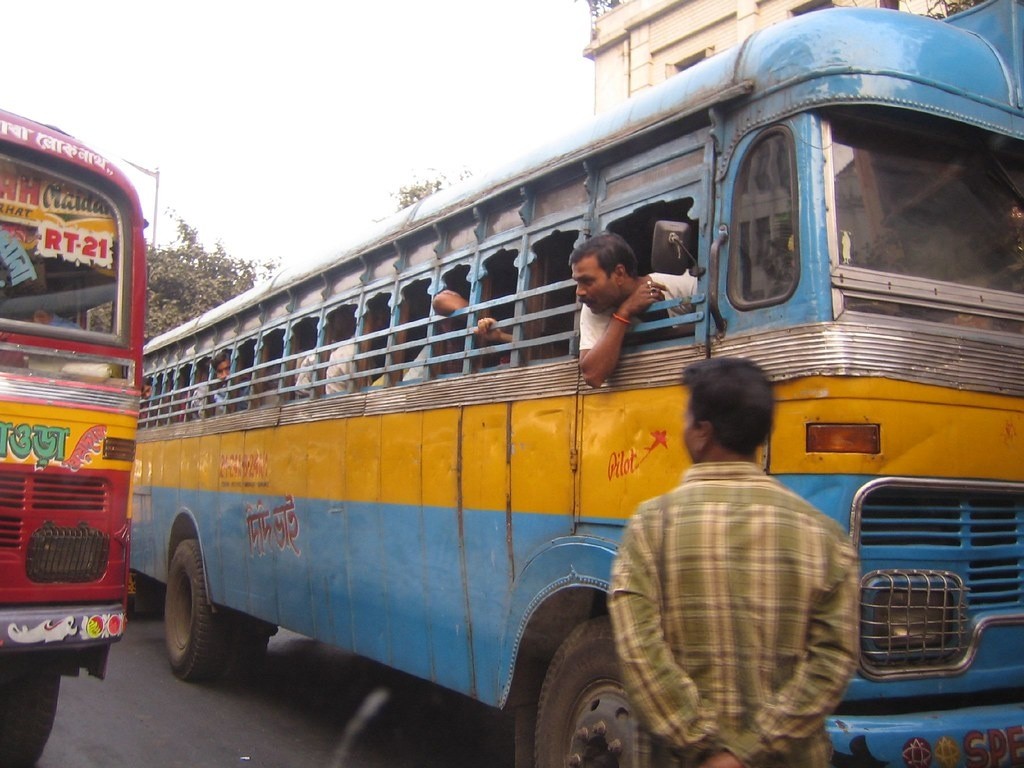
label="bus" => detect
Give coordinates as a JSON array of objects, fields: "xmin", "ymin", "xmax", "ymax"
[
  {"xmin": 0, "ymin": 105, "xmax": 152, "ymax": 768},
  {"xmin": 128, "ymin": 6, "xmax": 1024, "ymax": 767}
]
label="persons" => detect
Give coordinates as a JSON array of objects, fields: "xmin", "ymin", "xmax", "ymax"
[
  {"xmin": 570, "ymin": 234, "xmax": 697, "ymax": 389},
  {"xmin": 0, "ymin": 275, "xmax": 84, "ymax": 329},
  {"xmin": 606, "ymin": 357, "xmax": 861, "ymax": 768},
  {"xmin": 139, "ymin": 290, "xmax": 535, "ymax": 430}
]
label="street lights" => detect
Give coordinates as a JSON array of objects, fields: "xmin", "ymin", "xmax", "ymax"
[{"xmin": 119, "ymin": 156, "xmax": 162, "ymax": 250}]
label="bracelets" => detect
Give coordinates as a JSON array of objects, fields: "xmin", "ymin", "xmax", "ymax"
[{"xmin": 612, "ymin": 313, "xmax": 631, "ymax": 325}]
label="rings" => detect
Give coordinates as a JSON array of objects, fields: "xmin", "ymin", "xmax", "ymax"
[{"xmin": 647, "ymin": 280, "xmax": 656, "ymax": 296}]
